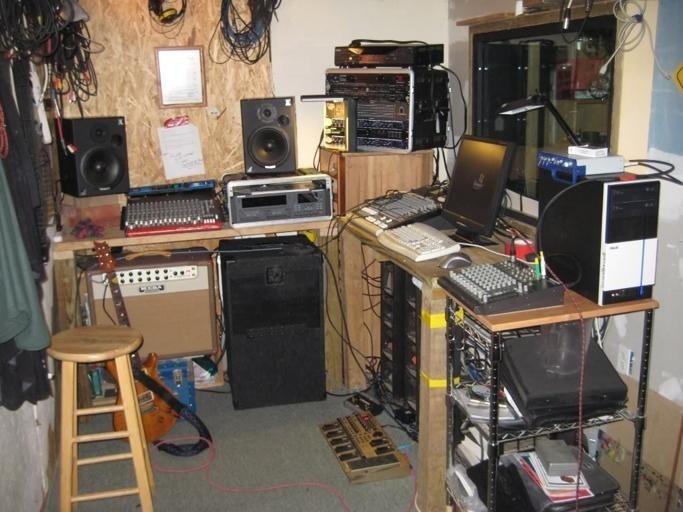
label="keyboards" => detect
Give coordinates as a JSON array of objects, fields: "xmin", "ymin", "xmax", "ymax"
[{"xmin": 376, "ymin": 221, "xmax": 461, "ymax": 262}]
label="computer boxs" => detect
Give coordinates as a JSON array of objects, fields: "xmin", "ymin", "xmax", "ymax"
[{"xmin": 536, "ymin": 167, "xmax": 661, "ymax": 307}]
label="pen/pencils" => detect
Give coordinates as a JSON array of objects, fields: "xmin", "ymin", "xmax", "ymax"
[{"xmin": 534, "ymin": 250, "xmax": 547, "ymax": 289}]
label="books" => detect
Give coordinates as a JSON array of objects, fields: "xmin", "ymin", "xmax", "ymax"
[{"xmin": 449, "ymin": 381, "xmax": 594, "ymax": 504}]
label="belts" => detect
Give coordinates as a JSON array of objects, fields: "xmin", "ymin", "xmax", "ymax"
[{"xmin": 14, "ymin": 57, "xmax": 55, "ymax": 264}]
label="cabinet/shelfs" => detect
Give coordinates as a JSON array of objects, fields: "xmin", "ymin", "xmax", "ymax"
[
  {"xmin": 457, "ymin": 0, "xmax": 658, "ymax": 227},
  {"xmin": 320, "ymin": 146, "xmax": 433, "ymax": 392}
]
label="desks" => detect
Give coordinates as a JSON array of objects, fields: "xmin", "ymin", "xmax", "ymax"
[
  {"xmin": 51, "ymin": 221, "xmax": 336, "ymax": 423},
  {"xmin": 339, "ymin": 220, "xmax": 659, "ymax": 512}
]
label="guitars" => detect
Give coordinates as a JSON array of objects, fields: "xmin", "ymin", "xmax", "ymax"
[{"xmin": 92, "ymin": 241, "xmax": 177, "ymax": 444}]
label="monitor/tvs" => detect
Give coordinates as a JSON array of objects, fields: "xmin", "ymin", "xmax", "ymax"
[{"xmin": 440, "ymin": 135, "xmax": 517, "ymax": 247}]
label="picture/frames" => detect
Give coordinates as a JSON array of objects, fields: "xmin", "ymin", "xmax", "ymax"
[{"xmin": 154, "ymin": 45, "xmax": 206, "ymax": 108}]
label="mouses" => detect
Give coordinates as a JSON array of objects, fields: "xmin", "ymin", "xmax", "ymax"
[{"xmin": 438, "ymin": 252, "xmax": 472, "ymax": 269}]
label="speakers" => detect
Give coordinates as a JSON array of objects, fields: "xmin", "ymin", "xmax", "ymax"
[
  {"xmin": 239, "ymin": 96, "xmax": 297, "ymax": 175},
  {"xmin": 218, "ymin": 234, "xmax": 326, "ymax": 410},
  {"xmin": 54, "ymin": 115, "xmax": 130, "ymax": 198}
]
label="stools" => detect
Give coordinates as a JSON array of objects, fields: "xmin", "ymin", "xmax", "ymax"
[{"xmin": 46, "ymin": 324, "xmax": 154, "ymax": 512}]
